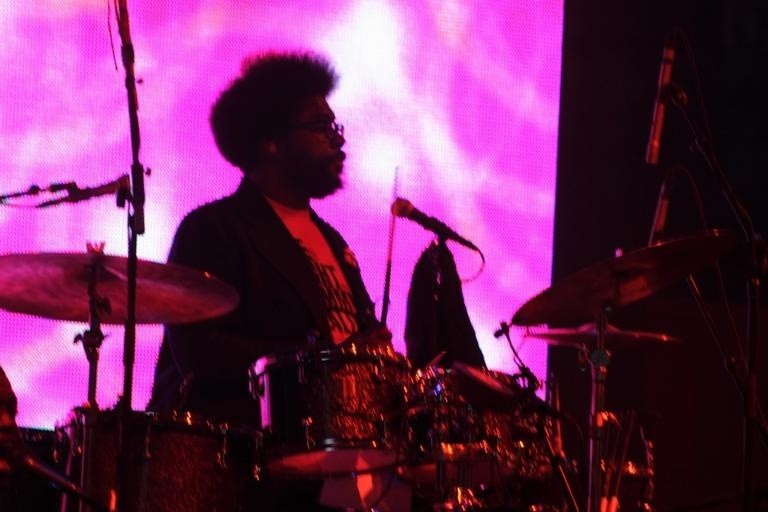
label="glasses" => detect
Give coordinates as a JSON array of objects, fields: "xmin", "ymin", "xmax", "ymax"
[{"xmin": 298, "ymin": 120, "xmax": 343, "ymax": 138}]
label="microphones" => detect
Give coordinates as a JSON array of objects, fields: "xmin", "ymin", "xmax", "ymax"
[
  {"xmin": 642, "ymin": 45, "xmax": 680, "ymax": 170},
  {"xmin": 389, "ymin": 196, "xmax": 479, "ymax": 255}
]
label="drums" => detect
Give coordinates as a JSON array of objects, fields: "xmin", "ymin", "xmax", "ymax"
[
  {"xmin": 57, "ymin": 408, "xmax": 233, "ymax": 510},
  {"xmin": 272, "ymin": 351, "xmax": 554, "ymax": 479}
]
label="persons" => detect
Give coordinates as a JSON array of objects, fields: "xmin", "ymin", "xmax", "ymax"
[{"xmin": 145, "ymin": 50, "xmax": 376, "ymax": 445}]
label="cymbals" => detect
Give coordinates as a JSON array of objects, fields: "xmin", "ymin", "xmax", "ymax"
[
  {"xmin": 512, "ymin": 233, "xmax": 704, "ymax": 345},
  {"xmin": 1, "ymin": 253, "xmax": 238, "ymax": 323}
]
